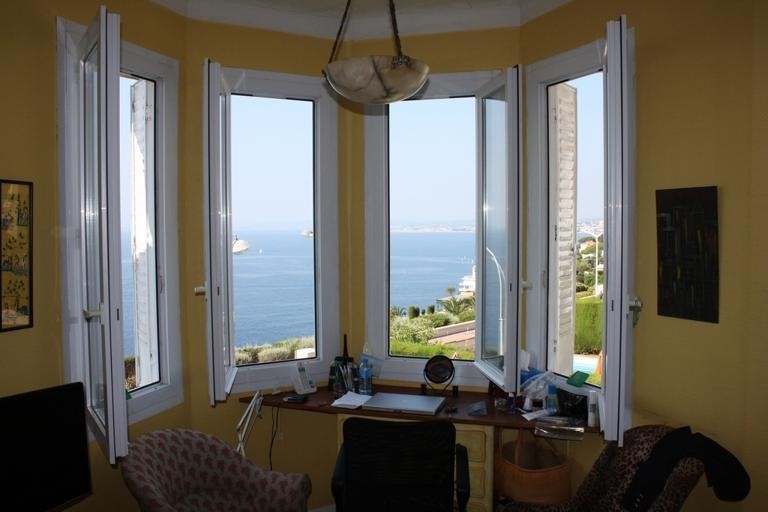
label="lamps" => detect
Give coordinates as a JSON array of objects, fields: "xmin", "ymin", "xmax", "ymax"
[{"xmin": 322, "ymin": 0, "xmax": 430, "ymax": 106}]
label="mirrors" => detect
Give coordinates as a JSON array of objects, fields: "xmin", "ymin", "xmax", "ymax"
[{"xmin": 420, "ymin": 355, "xmax": 458, "ymax": 397}]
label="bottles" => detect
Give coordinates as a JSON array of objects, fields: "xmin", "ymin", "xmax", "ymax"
[
  {"xmin": 545, "ymin": 383, "xmax": 562, "ymax": 416},
  {"xmin": 587, "ymin": 390, "xmax": 598, "ymax": 428},
  {"xmin": 327, "ymin": 356, "xmax": 359, "ymax": 393},
  {"xmin": 357, "ymin": 357, "xmax": 373, "ymax": 395}
]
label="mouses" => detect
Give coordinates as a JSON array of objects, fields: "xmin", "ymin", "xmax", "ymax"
[{"xmin": 446, "ymin": 406, "xmax": 458, "ymax": 414}]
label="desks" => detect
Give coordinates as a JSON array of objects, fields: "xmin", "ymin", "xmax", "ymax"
[{"xmin": 240, "ymin": 384, "xmax": 600, "ymax": 512}]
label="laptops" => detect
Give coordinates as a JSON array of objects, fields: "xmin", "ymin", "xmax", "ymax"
[{"xmin": 362, "ymin": 392, "xmax": 446, "ymax": 416}]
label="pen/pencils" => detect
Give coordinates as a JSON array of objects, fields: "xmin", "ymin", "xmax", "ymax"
[{"xmin": 335, "ymin": 363, "xmax": 353, "ymax": 388}]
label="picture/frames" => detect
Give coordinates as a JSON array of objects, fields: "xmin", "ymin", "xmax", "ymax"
[{"xmin": 0, "ymin": 180, "xmax": 33, "ymax": 332}]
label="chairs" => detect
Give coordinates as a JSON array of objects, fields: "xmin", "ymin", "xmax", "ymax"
[
  {"xmin": 331, "ymin": 416, "xmax": 470, "ymax": 512},
  {"xmin": 506, "ymin": 424, "xmax": 705, "ymax": 512},
  {"xmin": 122, "ymin": 429, "xmax": 311, "ymax": 512}
]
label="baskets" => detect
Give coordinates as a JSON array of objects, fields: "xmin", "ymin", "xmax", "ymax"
[{"xmin": 496, "ymin": 435, "xmax": 572, "ymax": 505}]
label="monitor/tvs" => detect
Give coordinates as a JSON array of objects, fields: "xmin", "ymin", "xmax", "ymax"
[{"xmin": 0, "ymin": 381, "xmax": 93, "ymax": 512}]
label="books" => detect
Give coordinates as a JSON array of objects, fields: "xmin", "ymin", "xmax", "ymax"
[{"xmin": 331, "ymin": 389, "xmax": 373, "ymax": 410}]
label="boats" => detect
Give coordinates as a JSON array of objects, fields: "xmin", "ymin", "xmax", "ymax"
[{"xmin": 301, "ymin": 229, "xmax": 312, "ymax": 238}]
